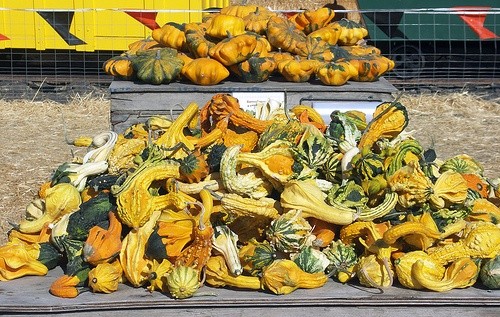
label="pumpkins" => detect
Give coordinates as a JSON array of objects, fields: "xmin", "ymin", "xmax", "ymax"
[
  {"xmin": 103, "ymin": 4, "xmax": 395, "ymax": 86},
  {"xmin": 0, "ymin": 94, "xmax": 500, "ymax": 299}
]
